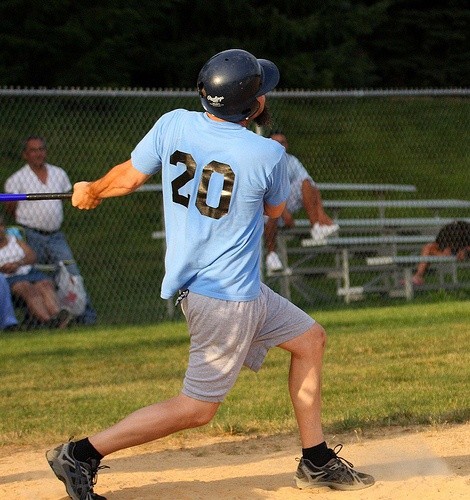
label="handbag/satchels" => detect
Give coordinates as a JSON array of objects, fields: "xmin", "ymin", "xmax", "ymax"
[{"xmin": 55, "ymin": 261, "xmax": 87, "ymax": 317}]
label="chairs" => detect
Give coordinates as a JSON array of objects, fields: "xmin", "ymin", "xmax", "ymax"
[{"xmin": 9, "ymin": 227, "xmax": 75, "ymax": 332}]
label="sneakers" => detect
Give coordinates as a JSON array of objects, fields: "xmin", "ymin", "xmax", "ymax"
[
  {"xmin": 45, "ymin": 436, "xmax": 110, "ymax": 500},
  {"xmin": 294, "ymin": 444, "xmax": 374, "ymax": 491}
]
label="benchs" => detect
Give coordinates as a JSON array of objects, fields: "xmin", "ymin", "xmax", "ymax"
[{"xmin": 152, "ymin": 183, "xmax": 470, "ymax": 302}]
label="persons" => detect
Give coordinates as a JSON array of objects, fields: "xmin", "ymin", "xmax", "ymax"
[
  {"xmin": 46, "ymin": 49, "xmax": 376, "ymax": 500},
  {"xmin": 0, "ymin": 218, "xmax": 70, "ymax": 330},
  {"xmin": 399, "ymin": 218, "xmax": 470, "ymax": 284},
  {"xmin": 2, "ymin": 136, "xmax": 96, "ymax": 325},
  {"xmin": 266, "ymin": 134, "xmax": 340, "ymax": 274}
]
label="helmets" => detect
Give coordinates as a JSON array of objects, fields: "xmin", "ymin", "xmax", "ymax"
[{"xmin": 197, "ymin": 49, "xmax": 280, "ymax": 124}]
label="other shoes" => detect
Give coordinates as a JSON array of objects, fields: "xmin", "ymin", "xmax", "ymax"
[
  {"xmin": 77, "ymin": 310, "xmax": 96, "ymax": 325},
  {"xmin": 43, "ymin": 318, "xmax": 59, "ymax": 329},
  {"xmin": 400, "ymin": 276, "xmax": 426, "ymax": 285},
  {"xmin": 266, "ymin": 251, "xmax": 283, "ymax": 271},
  {"xmin": 56, "ymin": 309, "xmax": 69, "ymax": 328},
  {"xmin": 311, "ymin": 222, "xmax": 340, "ymax": 241},
  {"xmin": 4, "ymin": 324, "xmax": 23, "ymax": 332}
]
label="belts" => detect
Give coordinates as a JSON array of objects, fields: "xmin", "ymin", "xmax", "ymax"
[{"xmin": 18, "ymin": 224, "xmax": 58, "ymax": 236}]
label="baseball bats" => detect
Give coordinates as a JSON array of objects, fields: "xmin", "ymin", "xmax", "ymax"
[{"xmin": 0, "ymin": 193, "xmax": 73, "ymax": 201}]
